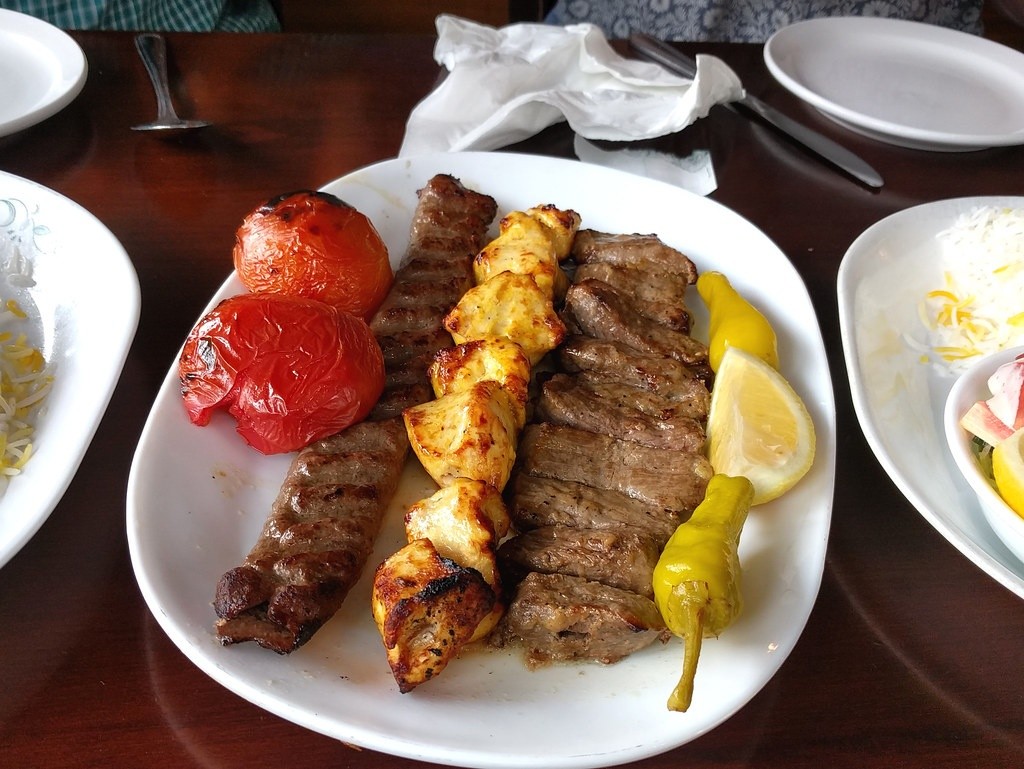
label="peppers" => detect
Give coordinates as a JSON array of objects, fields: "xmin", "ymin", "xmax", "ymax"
[
  {"xmin": 691, "ymin": 272, "xmax": 782, "ymax": 368},
  {"xmin": 653, "ymin": 475, "xmax": 755, "ymax": 711}
]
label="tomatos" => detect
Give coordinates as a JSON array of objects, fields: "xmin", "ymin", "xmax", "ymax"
[{"xmin": 181, "ymin": 193, "xmax": 393, "ymax": 451}]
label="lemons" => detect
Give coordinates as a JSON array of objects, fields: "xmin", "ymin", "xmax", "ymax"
[{"xmin": 703, "ymin": 345, "xmax": 815, "ymax": 504}]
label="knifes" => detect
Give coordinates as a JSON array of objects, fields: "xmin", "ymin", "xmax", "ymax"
[{"xmin": 631, "ymin": 24, "xmax": 883, "ymax": 188}]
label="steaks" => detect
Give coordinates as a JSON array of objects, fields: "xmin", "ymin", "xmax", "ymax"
[
  {"xmin": 214, "ymin": 176, "xmax": 497, "ymax": 655},
  {"xmin": 481, "ymin": 229, "xmax": 712, "ymax": 664}
]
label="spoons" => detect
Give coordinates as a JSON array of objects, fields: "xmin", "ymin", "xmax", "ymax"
[{"xmin": 128, "ymin": 32, "xmax": 208, "ymax": 137}]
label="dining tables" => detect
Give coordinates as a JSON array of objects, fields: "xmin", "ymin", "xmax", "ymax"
[{"xmin": 0, "ymin": 25, "xmax": 1023, "ymax": 768}]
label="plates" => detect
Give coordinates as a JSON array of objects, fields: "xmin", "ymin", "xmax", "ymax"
[
  {"xmin": 122, "ymin": 150, "xmax": 831, "ymax": 767},
  {"xmin": 0, "ymin": 8, "xmax": 89, "ymax": 141},
  {"xmin": 760, "ymin": 14, "xmax": 1024, "ymax": 156},
  {"xmin": 0, "ymin": 170, "xmax": 147, "ymax": 573},
  {"xmin": 831, "ymin": 191, "xmax": 1024, "ymax": 611}
]
